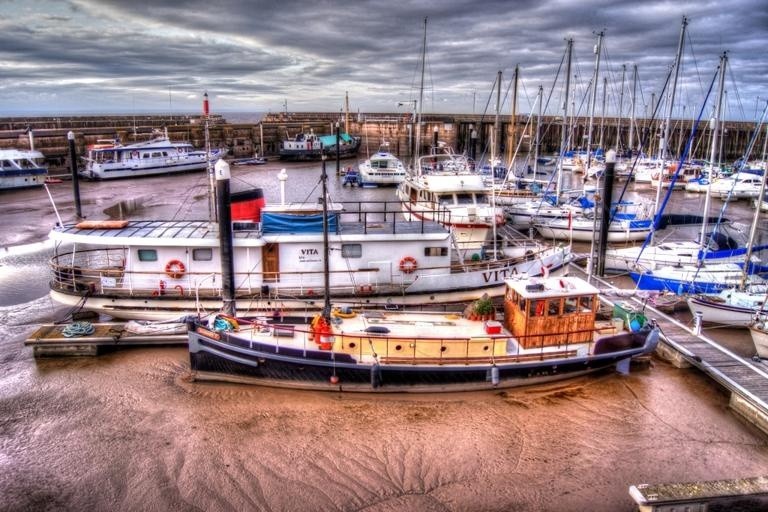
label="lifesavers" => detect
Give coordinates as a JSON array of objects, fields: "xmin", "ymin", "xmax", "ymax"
[
  {"xmin": 165, "ymin": 260, "xmax": 186, "ymax": 279},
  {"xmin": 400, "ymin": 256, "xmax": 418, "ymax": 274},
  {"xmin": 334, "ymin": 310, "xmax": 357, "ymax": 318}
]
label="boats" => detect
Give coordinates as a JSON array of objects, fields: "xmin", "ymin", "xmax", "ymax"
[
  {"xmin": 592, "ymin": 51, "xmax": 762, "ymax": 276},
  {"xmin": 530, "ymin": 16, "xmax": 693, "ymax": 235},
  {"xmin": 474, "ymin": 66, "xmax": 557, "ymax": 191},
  {"xmin": 485, "ymin": 85, "xmax": 585, "ymax": 206},
  {"xmin": 278, "ymin": 132, "xmax": 360, "ymax": 163},
  {"xmin": 342, "ymin": 166, "xmax": 361, "ymax": 187},
  {"xmin": 505, "ymin": 30, "xmax": 635, "ymax": 228},
  {"xmin": 685, "ymin": 156, "xmax": 768, "ymax": 328},
  {"xmin": 342, "ymin": 170, "xmax": 361, "ymax": 188},
  {"xmin": 81, "ymin": 137, "xmax": 229, "ymax": 180},
  {"xmin": 230, "ymin": 157, "xmax": 268, "ymax": 166},
  {"xmin": 537, "ymin": 63, "xmax": 697, "ymax": 183},
  {"xmin": 629, "ymin": 55, "xmax": 768, "ymax": 293},
  {"xmin": 278, "ymin": 124, "xmax": 362, "ymax": 163},
  {"xmin": 77, "ymin": 126, "xmax": 229, "ymax": 181},
  {"xmin": 747, "ymin": 311, "xmax": 768, "ymax": 360},
  {"xmin": 652, "ymin": 50, "xmax": 768, "ymax": 212}
]
